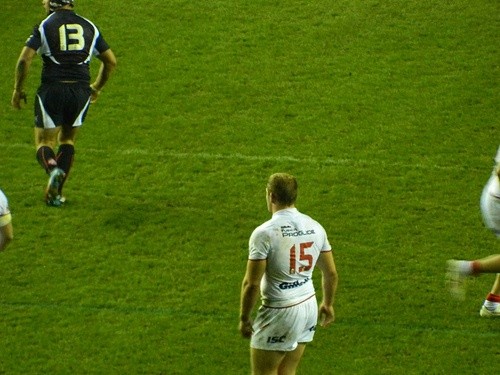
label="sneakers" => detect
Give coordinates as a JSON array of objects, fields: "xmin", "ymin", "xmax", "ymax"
[
  {"xmin": 446, "ymin": 260, "xmax": 467, "ymax": 303},
  {"xmin": 479, "ymin": 303, "xmax": 500, "ymax": 317},
  {"xmin": 44, "ymin": 169, "xmax": 66, "ymax": 207}
]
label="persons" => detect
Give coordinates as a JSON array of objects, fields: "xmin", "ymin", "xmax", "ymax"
[
  {"xmin": 0, "ymin": 190, "xmax": 13, "ymax": 254},
  {"xmin": 445, "ymin": 148, "xmax": 500, "ymax": 319},
  {"xmin": 11, "ymin": 0, "xmax": 117, "ymax": 207},
  {"xmin": 237, "ymin": 172, "xmax": 338, "ymax": 375}
]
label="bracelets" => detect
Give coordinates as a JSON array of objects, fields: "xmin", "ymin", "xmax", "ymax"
[{"xmin": 240, "ymin": 314, "xmax": 251, "ymax": 322}]
